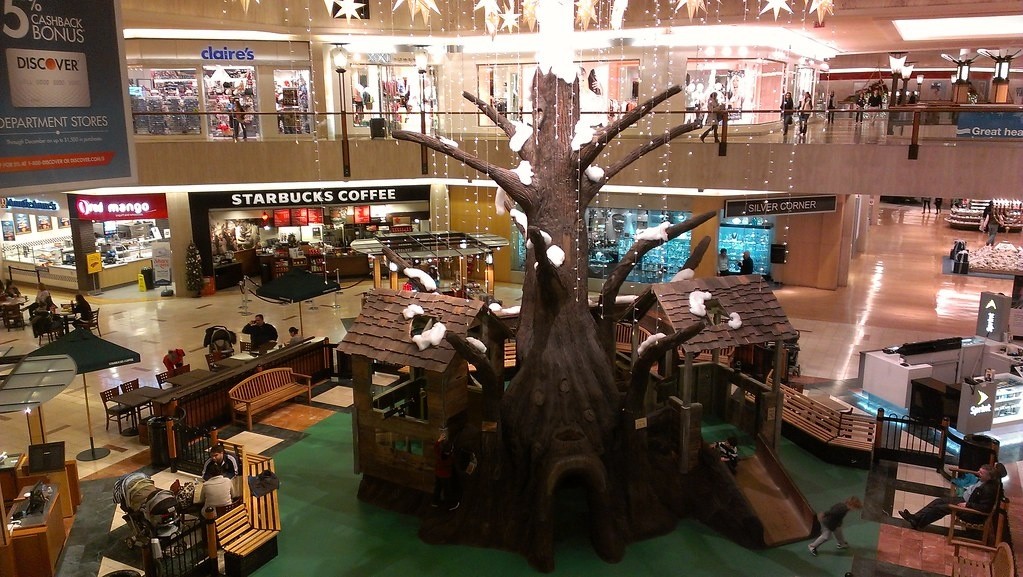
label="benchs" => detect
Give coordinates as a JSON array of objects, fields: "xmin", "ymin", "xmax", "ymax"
[
  {"xmin": 215, "ymin": 439, "xmax": 280, "ymax": 577},
  {"xmin": 228, "ymin": 369, "xmax": 313, "ymax": 432},
  {"xmin": 948, "ymin": 468, "xmax": 1015, "ymax": 577}
]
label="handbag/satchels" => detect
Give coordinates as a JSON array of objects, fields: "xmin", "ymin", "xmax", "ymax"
[{"xmin": 176, "ymin": 482, "xmax": 194, "ymax": 510}]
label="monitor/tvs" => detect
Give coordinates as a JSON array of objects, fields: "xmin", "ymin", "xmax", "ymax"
[{"xmin": 29, "ymin": 480, "xmax": 43, "ymax": 510}]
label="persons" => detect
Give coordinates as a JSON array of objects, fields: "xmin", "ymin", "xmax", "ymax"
[
  {"xmin": 923, "ymin": 197, "xmax": 942, "ymax": 214},
  {"xmin": 287, "ymin": 327, "xmax": 301, "ymax": 346},
  {"xmin": 779, "ymin": 92, "xmax": 813, "ymax": 135},
  {"xmin": 739, "ymin": 251, "xmax": 753, "ymax": 275},
  {"xmin": 0, "ymin": 279, "xmax": 21, "ymax": 300},
  {"xmin": 242, "ymin": 314, "xmax": 278, "ymax": 348},
  {"xmin": 827, "ymin": 94, "xmax": 835, "ymax": 123},
  {"xmin": 717, "ymin": 248, "xmax": 730, "ymax": 276},
  {"xmin": 29, "ymin": 283, "xmax": 64, "ymax": 336},
  {"xmin": 983, "ymin": 201, "xmax": 1003, "ymax": 246},
  {"xmin": 72, "ymin": 295, "xmax": 93, "ymax": 331},
  {"xmin": 855, "ymin": 89, "xmax": 915, "ymax": 124},
  {"xmin": 226, "ymin": 97, "xmax": 247, "ymax": 139},
  {"xmin": 808, "ymin": 496, "xmax": 863, "ymax": 556},
  {"xmin": 700, "ymin": 92, "xmax": 720, "ymax": 143},
  {"xmin": 276, "ymin": 97, "xmax": 283, "ymax": 128},
  {"xmin": 200, "ymin": 446, "xmax": 238, "ymax": 520},
  {"xmin": 898, "ymin": 466, "xmax": 997, "ymax": 530}
]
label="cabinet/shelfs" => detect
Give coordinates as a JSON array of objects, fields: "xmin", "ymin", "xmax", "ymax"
[{"xmin": 274, "ymin": 254, "xmax": 325, "ymax": 282}]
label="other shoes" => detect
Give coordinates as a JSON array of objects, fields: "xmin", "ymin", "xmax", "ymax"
[
  {"xmin": 700, "ymin": 136, "xmax": 705, "ymax": 143},
  {"xmin": 991, "ymin": 243, "xmax": 994, "ymax": 246},
  {"xmin": 837, "ymin": 543, "xmax": 849, "ymax": 549},
  {"xmin": 985, "ymin": 241, "xmax": 990, "ymax": 246},
  {"xmin": 808, "ymin": 544, "xmax": 817, "ymax": 556},
  {"xmin": 715, "ymin": 140, "xmax": 720, "ymax": 143},
  {"xmin": 928, "ymin": 212, "xmax": 931, "ymax": 213},
  {"xmin": 938, "ymin": 211, "xmax": 941, "ymax": 213},
  {"xmin": 935, "ymin": 212, "xmax": 938, "ymax": 214},
  {"xmin": 921, "ymin": 211, "xmax": 925, "ymax": 214}
]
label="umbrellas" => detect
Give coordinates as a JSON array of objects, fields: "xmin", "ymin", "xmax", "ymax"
[{"xmin": 256, "ymin": 268, "xmax": 342, "ymax": 337}]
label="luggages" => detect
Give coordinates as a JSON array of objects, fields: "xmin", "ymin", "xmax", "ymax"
[{"xmin": 370, "ymin": 117, "xmax": 386, "ymax": 140}]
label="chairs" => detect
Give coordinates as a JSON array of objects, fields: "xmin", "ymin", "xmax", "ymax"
[
  {"xmin": 100, "ymin": 342, "xmax": 276, "ymax": 433},
  {"xmin": 0, "ymin": 293, "xmax": 101, "ymax": 346}
]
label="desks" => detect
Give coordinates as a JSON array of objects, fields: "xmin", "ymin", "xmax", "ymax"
[
  {"xmin": 166, "ymin": 368, "xmax": 218, "ymax": 386},
  {"xmin": 214, "ymin": 353, "xmax": 261, "ymax": 370},
  {"xmin": 216, "ymin": 261, "xmax": 243, "ymax": 290},
  {"xmin": 111, "ymin": 386, "xmax": 167, "ymax": 435},
  {"xmin": 47, "ymin": 307, "xmax": 76, "ymax": 335},
  {"xmin": 0, "ymin": 297, "xmax": 29, "ymax": 324}
]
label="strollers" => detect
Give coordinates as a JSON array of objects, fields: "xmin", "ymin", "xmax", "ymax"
[
  {"xmin": 112, "ymin": 472, "xmax": 188, "ymax": 557},
  {"xmin": 204, "ymin": 325, "xmax": 236, "ymax": 370}
]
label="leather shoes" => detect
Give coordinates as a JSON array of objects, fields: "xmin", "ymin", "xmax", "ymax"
[
  {"xmin": 783, "ymin": 131, "xmax": 805, "ymax": 136},
  {"xmin": 898, "ymin": 509, "xmax": 921, "ymax": 529}
]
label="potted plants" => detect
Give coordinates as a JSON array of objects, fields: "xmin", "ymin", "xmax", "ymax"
[{"xmin": 185, "ymin": 240, "xmax": 204, "ymax": 298}]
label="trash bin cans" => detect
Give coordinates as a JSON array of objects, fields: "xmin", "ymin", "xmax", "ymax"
[
  {"xmin": 957, "ymin": 434, "xmax": 1000, "ymax": 479},
  {"xmin": 147, "ymin": 416, "xmax": 180, "ymax": 467},
  {"xmin": 141, "ymin": 269, "xmax": 154, "ymax": 289}
]
